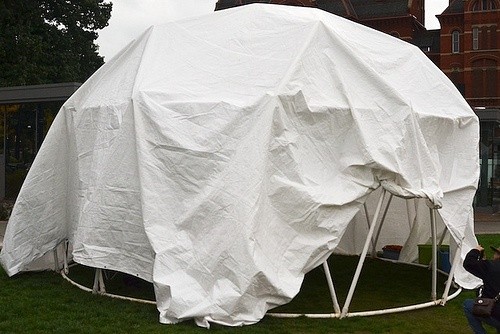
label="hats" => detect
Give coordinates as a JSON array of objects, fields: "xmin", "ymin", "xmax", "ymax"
[{"xmin": 490, "ymin": 246, "xmax": 500, "ymax": 254}]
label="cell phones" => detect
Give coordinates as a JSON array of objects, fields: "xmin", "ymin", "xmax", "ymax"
[{"xmin": 480, "ymin": 250, "xmax": 484, "ymax": 258}]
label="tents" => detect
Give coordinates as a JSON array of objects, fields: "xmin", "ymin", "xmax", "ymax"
[{"xmin": 0, "ymin": 3, "xmax": 484, "ymax": 330}]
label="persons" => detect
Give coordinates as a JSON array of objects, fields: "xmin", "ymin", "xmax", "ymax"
[{"xmin": 462, "ymin": 244, "xmax": 500, "ymax": 334}]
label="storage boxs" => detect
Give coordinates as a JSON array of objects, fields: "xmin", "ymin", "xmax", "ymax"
[
  {"xmin": 438, "ymin": 246, "xmax": 451, "ymax": 274},
  {"xmin": 382, "ymin": 245, "xmax": 403, "ymax": 259}
]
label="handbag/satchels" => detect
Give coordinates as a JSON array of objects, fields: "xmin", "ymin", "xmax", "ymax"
[{"xmin": 471, "ymin": 298, "xmax": 496, "ymax": 317}]
